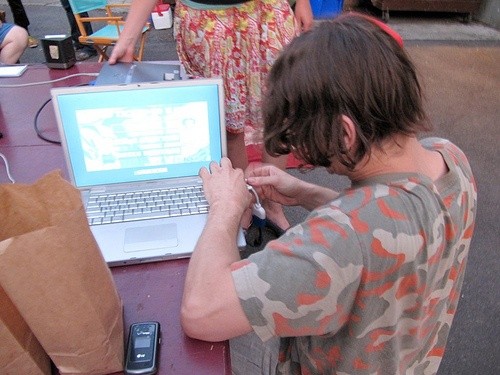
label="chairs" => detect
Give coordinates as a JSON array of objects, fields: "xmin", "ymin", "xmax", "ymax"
[{"xmin": 69, "ymin": 0, "xmax": 151, "ymax": 62}]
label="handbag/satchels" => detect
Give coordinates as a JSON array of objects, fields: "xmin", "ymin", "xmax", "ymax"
[{"xmin": 0, "ymin": 151, "xmax": 125, "ymax": 374}]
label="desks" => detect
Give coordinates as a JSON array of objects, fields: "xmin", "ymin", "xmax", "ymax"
[{"xmin": 0, "ymin": 58, "xmax": 249, "ymax": 375}]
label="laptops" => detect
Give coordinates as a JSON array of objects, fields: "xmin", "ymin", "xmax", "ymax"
[{"xmin": 49, "ymin": 77, "xmax": 248, "ymax": 267}]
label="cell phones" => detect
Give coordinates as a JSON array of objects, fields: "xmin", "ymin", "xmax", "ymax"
[
  {"xmin": 124, "ymin": 321, "xmax": 159, "ymax": 375},
  {"xmin": 0, "ymin": 66, "xmax": 28, "ymax": 77}
]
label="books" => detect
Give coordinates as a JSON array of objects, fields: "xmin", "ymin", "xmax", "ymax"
[{"xmin": 0, "ymin": 64, "xmax": 29, "ymax": 77}]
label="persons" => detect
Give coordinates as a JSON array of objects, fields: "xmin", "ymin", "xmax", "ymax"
[
  {"xmin": 108, "ymin": 0, "xmax": 317, "ymax": 238},
  {"xmin": 0, "ymin": 20, "xmax": 29, "ymax": 66},
  {"xmin": 180, "ymin": 18, "xmax": 476, "ymax": 375}
]
